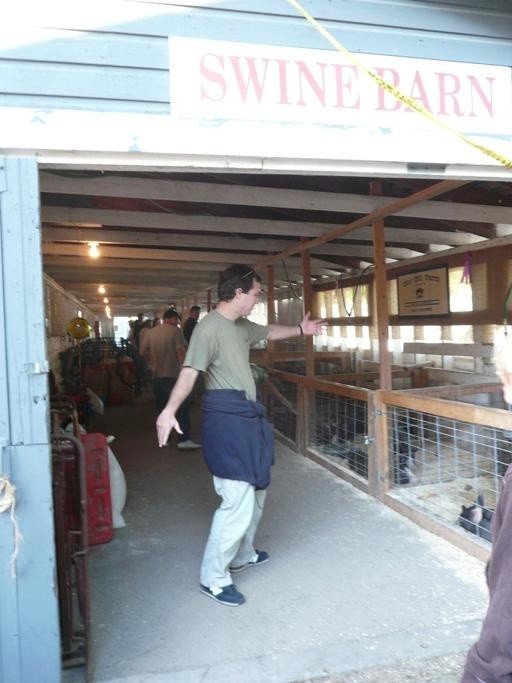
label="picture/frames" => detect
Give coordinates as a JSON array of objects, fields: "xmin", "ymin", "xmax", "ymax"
[{"xmin": 396, "ymin": 263, "xmax": 450, "ymax": 319}]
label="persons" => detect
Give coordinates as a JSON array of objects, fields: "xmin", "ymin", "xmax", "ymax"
[
  {"xmin": 124, "ymin": 302, "xmax": 205, "ymax": 449},
  {"xmin": 462, "ymin": 290, "xmax": 512, "ymax": 683},
  {"xmin": 155, "ymin": 263, "xmax": 330, "ymax": 606}
]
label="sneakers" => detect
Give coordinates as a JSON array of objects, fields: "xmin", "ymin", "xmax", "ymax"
[
  {"xmin": 199, "ymin": 580, "xmax": 246, "ymax": 607},
  {"xmin": 229, "ymin": 547, "xmax": 270, "ymax": 572},
  {"xmin": 176, "ymin": 439, "xmax": 202, "ymax": 449}
]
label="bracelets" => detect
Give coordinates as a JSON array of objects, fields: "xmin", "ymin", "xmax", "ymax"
[{"xmin": 297, "ymin": 323, "xmax": 304, "ymax": 335}]
